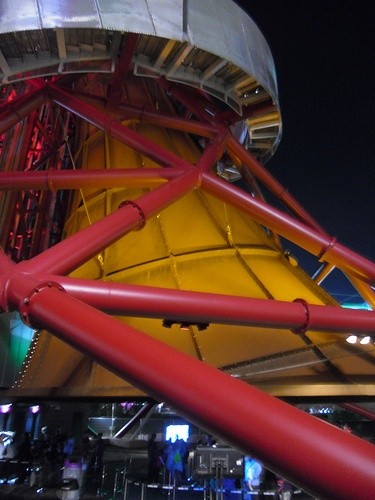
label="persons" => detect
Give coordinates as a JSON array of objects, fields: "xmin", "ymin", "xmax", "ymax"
[
  {"xmin": 0, "ymin": 419, "xmax": 106, "ymax": 495},
  {"xmin": 148, "ymin": 433, "xmax": 212, "ymax": 496}
]
